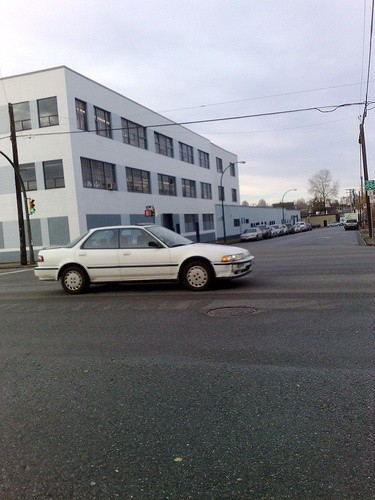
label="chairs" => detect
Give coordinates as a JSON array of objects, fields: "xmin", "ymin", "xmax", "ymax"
[
  {"xmin": 99, "ymin": 238, "xmax": 108, "ymax": 247},
  {"xmin": 137, "ymin": 235, "xmax": 148, "ymax": 246}
]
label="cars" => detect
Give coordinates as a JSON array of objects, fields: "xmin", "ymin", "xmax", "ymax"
[
  {"xmin": 33, "ymin": 223, "xmax": 257, "ymax": 295},
  {"xmin": 327, "ymin": 222, "xmax": 344, "ymax": 228},
  {"xmin": 240, "ymin": 221, "xmax": 312, "ymax": 242}
]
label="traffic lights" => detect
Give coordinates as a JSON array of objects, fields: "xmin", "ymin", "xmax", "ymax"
[
  {"xmin": 145, "ymin": 210, "xmax": 152, "ymax": 217},
  {"xmin": 29, "ymin": 198, "xmax": 36, "ymax": 214}
]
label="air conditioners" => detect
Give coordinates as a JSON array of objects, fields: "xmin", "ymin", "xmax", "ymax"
[{"xmin": 107, "ymin": 183, "xmax": 113, "ymax": 190}]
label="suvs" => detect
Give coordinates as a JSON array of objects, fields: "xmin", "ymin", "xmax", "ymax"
[{"xmin": 344, "ymin": 219, "xmax": 359, "ymax": 230}]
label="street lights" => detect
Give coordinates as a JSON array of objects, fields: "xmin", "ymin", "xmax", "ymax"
[
  {"xmin": 220, "ymin": 160, "xmax": 245, "ymax": 244},
  {"xmin": 281, "ymin": 188, "xmax": 297, "ymax": 223}
]
